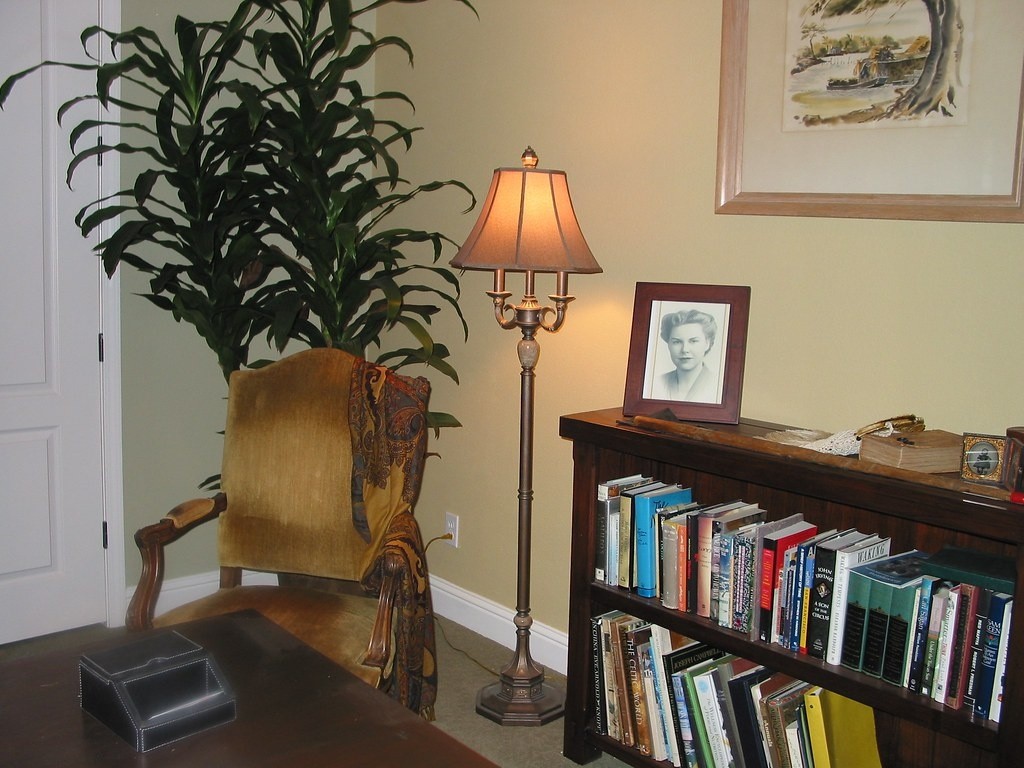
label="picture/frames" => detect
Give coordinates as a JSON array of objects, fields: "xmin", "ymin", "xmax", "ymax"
[
  {"xmin": 713, "ymin": 0, "xmax": 1024, "ymax": 224},
  {"xmin": 621, "ymin": 281, "xmax": 751, "ymax": 426}
]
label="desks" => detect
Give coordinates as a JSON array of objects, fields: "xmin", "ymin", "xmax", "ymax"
[{"xmin": 0, "ymin": 605, "xmax": 501, "ymax": 768}]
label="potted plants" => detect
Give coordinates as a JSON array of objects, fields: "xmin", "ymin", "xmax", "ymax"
[{"xmin": 0, "ymin": 0, "xmax": 478, "ymax": 601}]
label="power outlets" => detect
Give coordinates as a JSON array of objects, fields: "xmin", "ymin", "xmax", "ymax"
[{"xmin": 443, "ymin": 511, "xmax": 459, "ymax": 549}]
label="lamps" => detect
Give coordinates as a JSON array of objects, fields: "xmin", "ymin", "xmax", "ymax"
[{"xmin": 449, "ymin": 147, "xmax": 604, "ymax": 726}]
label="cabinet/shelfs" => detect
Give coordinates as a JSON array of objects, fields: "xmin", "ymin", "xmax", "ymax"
[{"xmin": 559, "ymin": 407, "xmax": 1024, "ymax": 768}]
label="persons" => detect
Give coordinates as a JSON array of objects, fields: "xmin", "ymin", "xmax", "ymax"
[
  {"xmin": 973, "ymin": 448, "xmax": 992, "ymax": 474},
  {"xmin": 660, "ymin": 309, "xmax": 718, "ymax": 401}
]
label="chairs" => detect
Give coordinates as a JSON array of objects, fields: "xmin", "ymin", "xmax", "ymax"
[{"xmin": 127, "ymin": 346, "xmax": 439, "ymax": 709}]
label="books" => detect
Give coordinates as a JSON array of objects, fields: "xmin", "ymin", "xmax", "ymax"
[
  {"xmin": 592, "ymin": 474, "xmax": 1016, "ymax": 768},
  {"xmin": 859, "ymin": 429, "xmax": 964, "ymax": 474}
]
